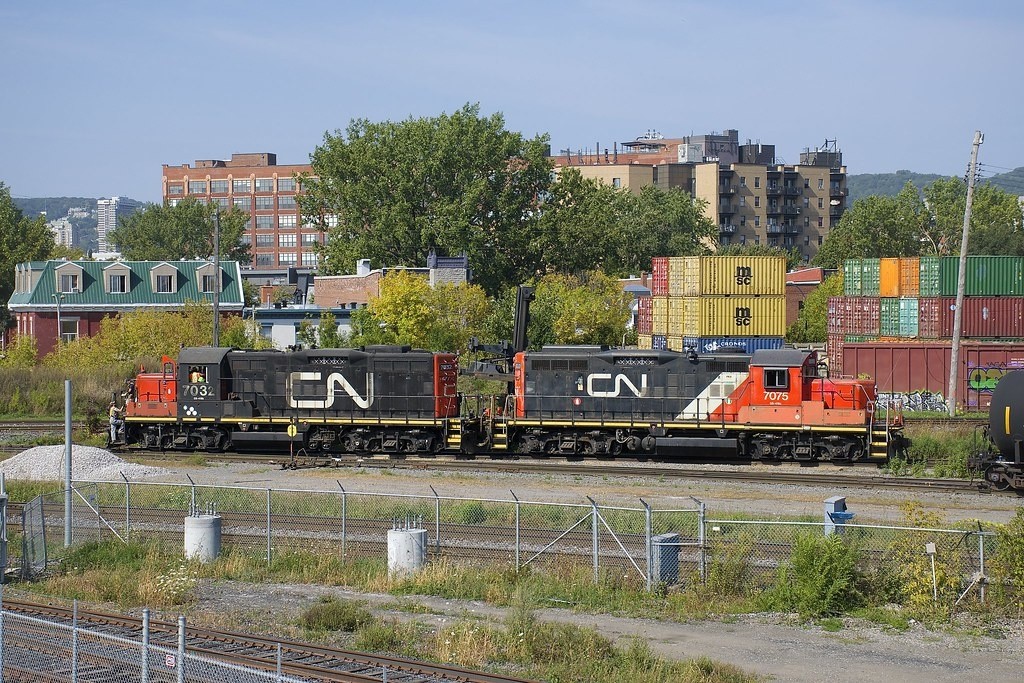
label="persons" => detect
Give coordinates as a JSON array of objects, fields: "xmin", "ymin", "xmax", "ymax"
[
  {"xmin": 124, "ymin": 378, "xmax": 134, "ymax": 403},
  {"xmin": 110, "ymin": 400, "xmax": 124, "ymax": 443},
  {"xmin": 188, "ymin": 366, "xmax": 206, "ymax": 383}
]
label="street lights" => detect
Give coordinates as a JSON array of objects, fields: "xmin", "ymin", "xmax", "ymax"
[
  {"xmin": 210, "ymin": 207, "xmax": 220, "ymax": 347},
  {"xmin": 949, "ymin": 130, "xmax": 984, "ymax": 418},
  {"xmin": 52, "ymin": 294, "xmax": 65, "ymax": 342}
]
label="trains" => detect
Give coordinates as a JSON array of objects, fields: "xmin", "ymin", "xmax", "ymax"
[
  {"xmin": 965, "ymin": 370, "xmax": 1023, "ymax": 497},
  {"xmin": 105, "ymin": 347, "xmax": 913, "ymax": 463}
]
